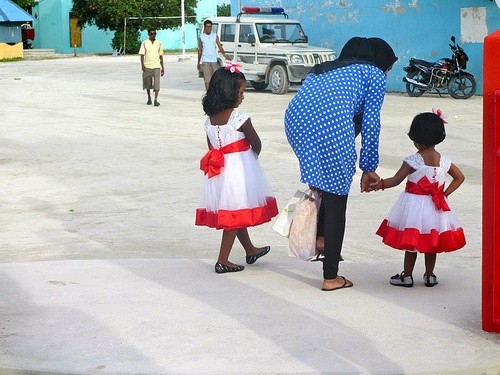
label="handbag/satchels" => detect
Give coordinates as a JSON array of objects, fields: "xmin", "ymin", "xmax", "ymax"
[{"xmin": 272, "ymin": 188, "xmax": 320, "ymax": 262}]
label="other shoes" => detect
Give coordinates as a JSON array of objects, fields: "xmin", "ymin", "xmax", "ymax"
[
  {"xmin": 147, "ymin": 100, "xmax": 152, "ymax": 105},
  {"xmin": 215, "ymin": 263, "xmax": 244, "ymax": 273},
  {"xmin": 246, "ymin": 246, "xmax": 270, "ymax": 264},
  {"xmin": 154, "ymin": 100, "xmax": 160, "ymax": 106},
  {"xmin": 423, "ymin": 272, "xmax": 438, "ymax": 287},
  {"xmin": 390, "ymin": 271, "xmax": 413, "ymax": 287}
]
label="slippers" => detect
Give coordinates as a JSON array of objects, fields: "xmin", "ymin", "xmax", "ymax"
[
  {"xmin": 322, "ymin": 276, "xmax": 353, "ymax": 290},
  {"xmin": 311, "ymin": 252, "xmax": 344, "ymax": 261}
]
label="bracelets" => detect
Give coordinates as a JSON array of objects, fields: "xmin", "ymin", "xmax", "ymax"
[{"xmin": 381, "ymin": 179, "xmax": 385, "ymax": 191}]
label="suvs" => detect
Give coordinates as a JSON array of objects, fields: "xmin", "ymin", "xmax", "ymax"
[{"xmin": 197, "ymin": 6, "xmax": 338, "ymax": 95}]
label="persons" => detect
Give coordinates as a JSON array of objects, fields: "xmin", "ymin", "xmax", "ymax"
[
  {"xmin": 197, "ymin": 20, "xmax": 229, "ymax": 92},
  {"xmin": 284, "ymin": 36, "xmax": 398, "ymax": 292},
  {"xmin": 365, "ymin": 109, "xmax": 467, "ymax": 287},
  {"xmin": 139, "ymin": 28, "xmax": 164, "ymax": 106},
  {"xmin": 195, "ymin": 62, "xmax": 279, "ymax": 274}
]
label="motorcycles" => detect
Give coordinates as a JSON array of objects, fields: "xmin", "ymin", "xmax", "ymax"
[{"xmin": 402, "ymin": 35, "xmax": 476, "ymax": 100}]
